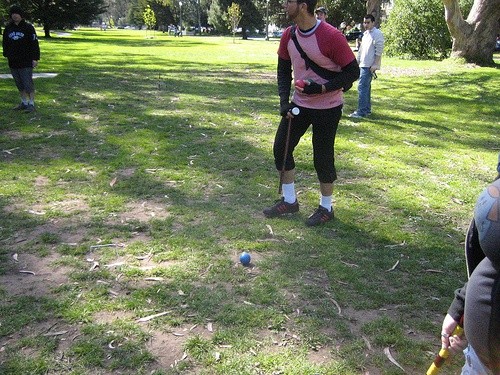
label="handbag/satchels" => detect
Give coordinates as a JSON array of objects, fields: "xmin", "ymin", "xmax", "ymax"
[{"xmin": 290, "ymin": 25, "xmax": 353, "ymax": 92}]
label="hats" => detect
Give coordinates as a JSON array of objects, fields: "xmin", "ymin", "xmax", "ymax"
[{"xmin": 315, "ymin": 7, "xmax": 327, "ymax": 14}]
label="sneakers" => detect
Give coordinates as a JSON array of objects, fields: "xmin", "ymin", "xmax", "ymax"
[
  {"xmin": 263, "ymin": 197, "xmax": 299, "ymax": 215},
  {"xmin": 306, "ymin": 205, "xmax": 334, "ymax": 226}
]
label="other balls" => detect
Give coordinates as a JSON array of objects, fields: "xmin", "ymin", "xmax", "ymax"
[
  {"xmin": 295, "ymin": 79, "xmax": 312, "ymax": 88},
  {"xmin": 239, "ymin": 252, "xmax": 251, "ymax": 264}
]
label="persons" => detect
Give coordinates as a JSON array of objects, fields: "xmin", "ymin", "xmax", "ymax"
[
  {"xmin": 314, "ymin": 7, "xmax": 327, "ymax": 23},
  {"xmin": 345, "ymin": 14, "xmax": 384, "ymax": 118},
  {"xmin": 3, "ymin": 6, "xmax": 40, "ymax": 113},
  {"xmin": 262, "ymin": 0, "xmax": 360, "ymax": 228},
  {"xmin": 441, "ymin": 153, "xmax": 500, "ymax": 375}
]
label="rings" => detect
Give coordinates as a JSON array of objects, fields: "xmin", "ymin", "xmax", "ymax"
[{"xmin": 441, "ymin": 332, "xmax": 449, "ymax": 338}]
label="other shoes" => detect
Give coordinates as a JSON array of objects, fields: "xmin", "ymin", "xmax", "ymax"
[
  {"xmin": 13, "ymin": 102, "xmax": 29, "ymax": 110},
  {"xmin": 348, "ymin": 111, "xmax": 371, "ymax": 118},
  {"xmin": 25, "ymin": 103, "xmax": 35, "ymax": 113}
]
